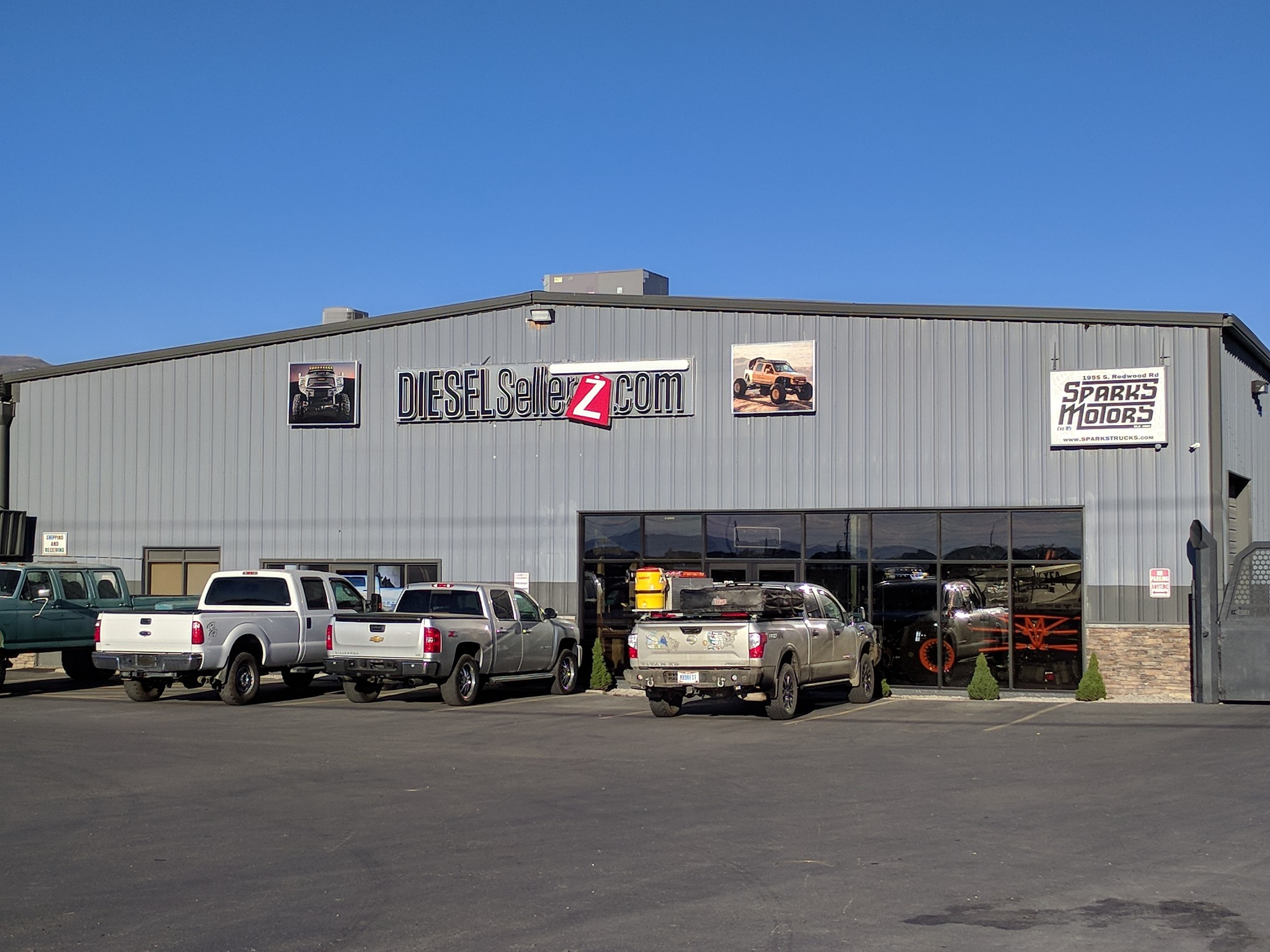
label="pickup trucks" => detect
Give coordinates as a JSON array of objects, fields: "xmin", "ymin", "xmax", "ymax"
[
  {"xmin": 622, "ymin": 579, "xmax": 884, "ymax": 721},
  {"xmin": 323, "ymin": 580, "xmax": 584, "ymax": 708},
  {"xmin": 870, "ymin": 571, "xmax": 1013, "ymax": 674},
  {"xmin": 92, "ymin": 566, "xmax": 381, "ymax": 708},
  {"xmin": 0, "ymin": 562, "xmax": 202, "ymax": 687}
]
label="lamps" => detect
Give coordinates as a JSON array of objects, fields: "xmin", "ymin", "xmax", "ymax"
[
  {"xmin": 530, "ymin": 308, "xmax": 556, "ymax": 325},
  {"xmin": 1251, "ymin": 380, "xmax": 1269, "ymax": 395}
]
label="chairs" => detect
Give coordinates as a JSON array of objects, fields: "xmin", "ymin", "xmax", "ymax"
[
  {"xmin": 7, "ymin": 573, "xmax": 30, "ymax": 600},
  {"xmin": 64, "ymin": 581, "xmax": 86, "ymax": 599},
  {"xmin": 98, "ymin": 580, "xmax": 118, "ymax": 599}
]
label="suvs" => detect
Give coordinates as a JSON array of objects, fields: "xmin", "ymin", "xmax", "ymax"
[
  {"xmin": 733, "ymin": 357, "xmax": 813, "ymax": 405},
  {"xmin": 292, "ymin": 365, "xmax": 351, "ymax": 422}
]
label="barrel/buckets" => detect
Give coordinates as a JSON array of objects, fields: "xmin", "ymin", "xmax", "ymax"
[{"xmin": 635, "ymin": 567, "xmax": 665, "ymax": 609}]
